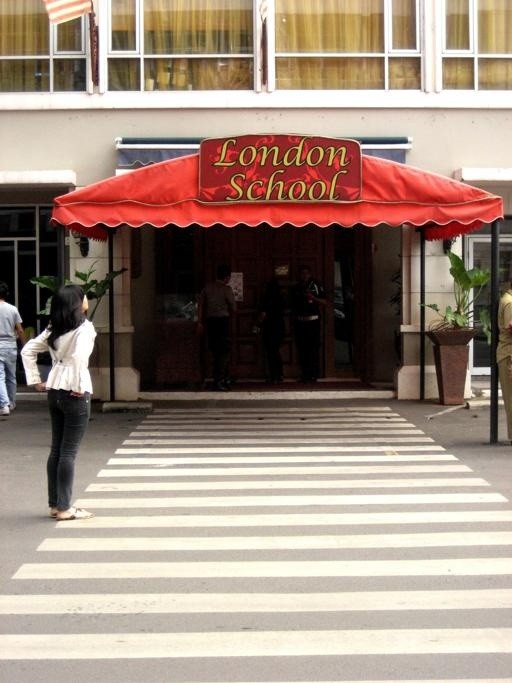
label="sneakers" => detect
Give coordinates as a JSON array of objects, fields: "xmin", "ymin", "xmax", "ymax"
[{"xmin": 0, "ymin": 402, "xmax": 17, "ymax": 416}]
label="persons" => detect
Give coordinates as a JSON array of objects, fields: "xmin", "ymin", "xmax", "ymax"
[
  {"xmin": 291, "ymin": 262, "xmax": 331, "ymax": 384},
  {"xmin": 19, "ymin": 279, "xmax": 97, "ymax": 518},
  {"xmin": 1, "ymin": 295, "xmax": 28, "ymax": 418},
  {"xmin": 195, "ymin": 263, "xmax": 238, "ymax": 390},
  {"xmin": 252, "ymin": 274, "xmax": 291, "ymax": 388},
  {"xmin": 497, "ymin": 288, "xmax": 512, "ymax": 440}
]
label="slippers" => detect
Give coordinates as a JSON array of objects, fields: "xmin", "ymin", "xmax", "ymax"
[{"xmin": 50, "ymin": 506, "xmax": 96, "ymax": 521}]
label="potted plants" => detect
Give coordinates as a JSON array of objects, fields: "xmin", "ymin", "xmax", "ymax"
[{"xmin": 418, "ymin": 248, "xmax": 506, "ymax": 405}]
